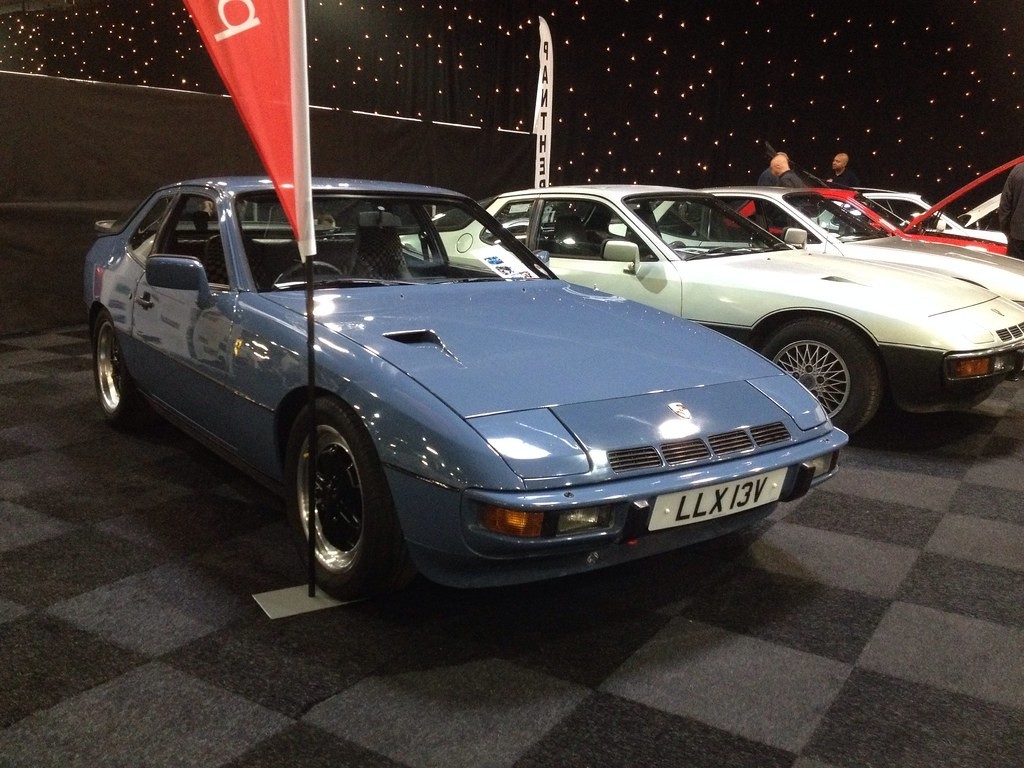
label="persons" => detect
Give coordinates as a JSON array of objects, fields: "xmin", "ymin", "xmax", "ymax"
[
  {"xmin": 756, "ymin": 152, "xmax": 858, "ymax": 238},
  {"xmin": 998, "ymin": 161, "xmax": 1024, "ymax": 262}
]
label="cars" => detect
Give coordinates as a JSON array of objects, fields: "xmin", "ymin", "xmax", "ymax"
[{"xmin": 573, "ymin": 185, "xmax": 1023, "ymax": 306}]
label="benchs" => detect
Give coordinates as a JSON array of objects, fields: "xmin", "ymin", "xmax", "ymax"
[{"xmin": 176, "ymin": 239, "xmax": 353, "ymax": 273}]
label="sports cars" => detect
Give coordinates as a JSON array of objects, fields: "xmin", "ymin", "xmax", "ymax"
[
  {"xmin": 81, "ymin": 176, "xmax": 852, "ymax": 588},
  {"xmin": 390, "ymin": 182, "xmax": 1023, "ymax": 436}
]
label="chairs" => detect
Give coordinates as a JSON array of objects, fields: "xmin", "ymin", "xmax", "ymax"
[
  {"xmin": 334, "ymin": 227, "xmax": 412, "ymax": 280},
  {"xmin": 201, "ymin": 234, "xmax": 275, "ymax": 289},
  {"xmin": 626, "ymin": 203, "xmax": 662, "ymax": 261},
  {"xmin": 547, "ymin": 212, "xmax": 597, "ymax": 257}
]
